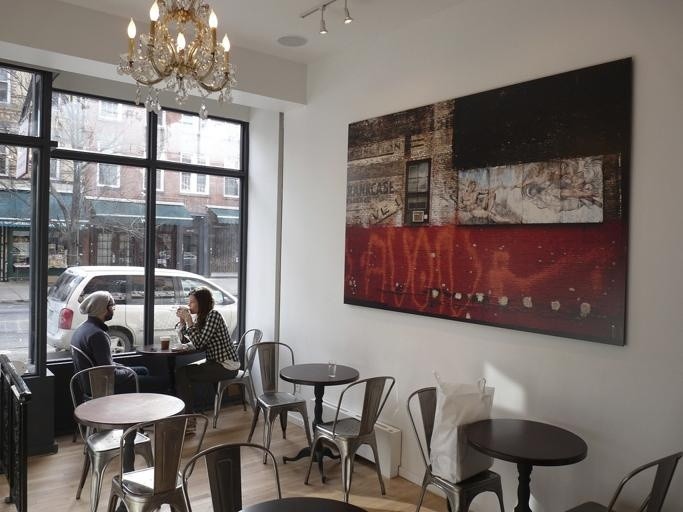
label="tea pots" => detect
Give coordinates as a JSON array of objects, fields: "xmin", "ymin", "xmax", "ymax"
[{"xmin": 167, "ymin": 329, "xmax": 182, "ymax": 349}]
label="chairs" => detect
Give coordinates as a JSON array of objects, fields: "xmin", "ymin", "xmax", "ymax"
[
  {"xmin": 106, "ymin": 415, "xmax": 208, "ymax": 512},
  {"xmin": 404, "ymin": 384, "xmax": 504, "ymax": 512},
  {"xmin": 244, "ymin": 339, "xmax": 314, "ymax": 465},
  {"xmin": 177, "ymin": 443, "xmax": 285, "ymax": 512},
  {"xmin": 566, "ymin": 447, "xmax": 681, "ymax": 511},
  {"xmin": 304, "ymin": 377, "xmax": 396, "ymax": 504},
  {"xmin": 207, "ymin": 324, "xmax": 266, "ymax": 429},
  {"xmin": 69, "ymin": 366, "xmax": 160, "ymax": 512},
  {"xmin": 70, "ymin": 344, "xmax": 141, "ymax": 445}
]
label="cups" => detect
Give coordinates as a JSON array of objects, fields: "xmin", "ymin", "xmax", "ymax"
[
  {"xmin": 328, "ymin": 360, "xmax": 337, "ymax": 377},
  {"xmin": 160, "ymin": 336, "xmax": 171, "ymax": 350}
]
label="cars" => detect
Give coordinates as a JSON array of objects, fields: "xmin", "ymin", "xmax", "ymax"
[
  {"xmin": 160, "ymin": 250, "xmax": 197, "ymax": 266},
  {"xmin": 13, "ymin": 243, "xmax": 29, "ymax": 264}
]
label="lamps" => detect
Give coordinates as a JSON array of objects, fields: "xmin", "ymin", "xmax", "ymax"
[
  {"xmin": 116, "ymin": 1, "xmax": 241, "ymax": 119},
  {"xmin": 296, "ymin": 0, "xmax": 353, "ymax": 35}
]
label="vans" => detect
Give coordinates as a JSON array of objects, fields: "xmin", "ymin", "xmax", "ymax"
[{"xmin": 46, "ymin": 265, "xmax": 240, "ymax": 355}]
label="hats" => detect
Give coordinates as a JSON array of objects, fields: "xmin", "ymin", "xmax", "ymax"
[{"xmin": 79, "ymin": 292, "xmax": 109, "ymax": 314}]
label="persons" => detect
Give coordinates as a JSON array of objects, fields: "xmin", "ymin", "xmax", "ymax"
[
  {"xmin": 521, "ymin": 163, "xmax": 552, "ymax": 194},
  {"xmin": 174, "ymin": 289, "xmax": 240, "ymax": 414},
  {"xmin": 69, "ymin": 290, "xmax": 148, "ymax": 398},
  {"xmin": 455, "ymin": 179, "xmax": 496, "ymax": 222},
  {"xmin": 560, "ymin": 171, "xmax": 593, "ymax": 199}
]
